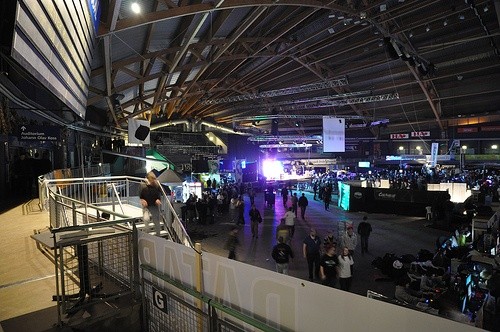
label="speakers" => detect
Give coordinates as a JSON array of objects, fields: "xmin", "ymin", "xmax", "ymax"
[{"xmin": 135, "ymin": 124, "xmax": 149, "ymax": 141}]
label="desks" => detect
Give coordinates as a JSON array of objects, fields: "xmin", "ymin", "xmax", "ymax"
[{"xmin": 31, "ymin": 227, "xmax": 130, "ymax": 315}]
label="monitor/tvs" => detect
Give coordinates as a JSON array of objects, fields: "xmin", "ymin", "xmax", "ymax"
[{"xmin": 464, "ymin": 272, "xmax": 471, "ymax": 287}]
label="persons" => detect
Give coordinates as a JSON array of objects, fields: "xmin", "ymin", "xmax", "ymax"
[
  {"xmin": 298, "ymin": 193, "xmax": 308, "ymax": 219},
  {"xmin": 425, "ymin": 204, "xmax": 432, "ymax": 219},
  {"xmin": 457, "ymin": 230, "xmax": 471, "ymax": 246},
  {"xmin": 318, "ymin": 167, "xmax": 500, "ymax": 201},
  {"xmin": 394, "ymin": 277, "xmax": 435, "ymax": 304},
  {"xmin": 224, "ymin": 227, "xmax": 242, "ymax": 260},
  {"xmin": 140, "ymin": 172, "xmax": 162, "ymax": 238},
  {"xmin": 302, "ymin": 226, "xmax": 357, "ymax": 291},
  {"xmin": 265, "ymin": 187, "xmax": 288, "ymax": 208},
  {"xmin": 249, "ymin": 204, "xmax": 260, "ymax": 240},
  {"xmin": 248, "ymin": 188, "xmax": 256, "ymax": 205},
  {"xmin": 173, "ymin": 185, "xmax": 245, "ymax": 226},
  {"xmin": 290, "ymin": 193, "xmax": 298, "ymax": 218},
  {"xmin": 313, "ymin": 183, "xmax": 332, "ymax": 210},
  {"xmin": 276, "ymin": 219, "xmax": 290, "ymax": 245},
  {"xmin": 358, "ymin": 216, "xmax": 372, "ymax": 256},
  {"xmin": 284, "ymin": 207, "xmax": 297, "ymax": 237},
  {"xmin": 272, "ymin": 236, "xmax": 294, "ymax": 275},
  {"xmin": 102, "ymin": 212, "xmax": 110, "ymax": 220}
]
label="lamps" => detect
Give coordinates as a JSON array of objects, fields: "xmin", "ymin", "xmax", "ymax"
[{"xmin": 113, "ymin": 93, "xmax": 125, "ymax": 101}]
